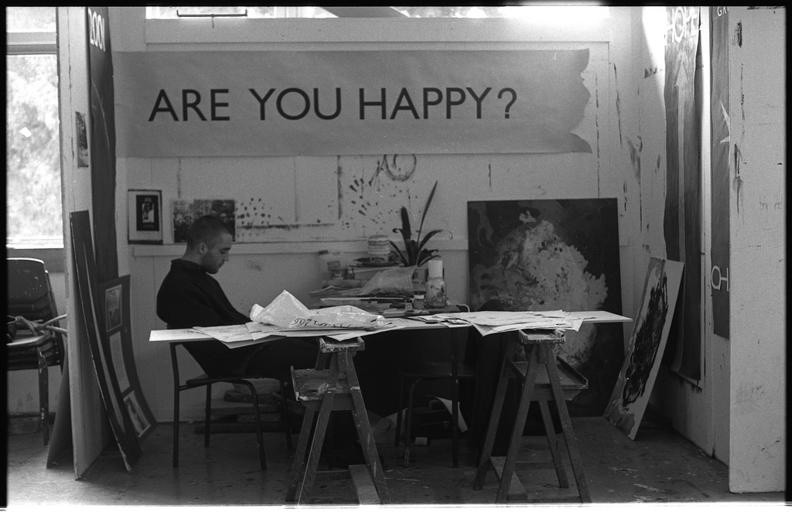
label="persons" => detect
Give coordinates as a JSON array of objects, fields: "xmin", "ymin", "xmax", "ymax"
[
  {"xmin": 142, "ymin": 201, "xmax": 154, "ymax": 223},
  {"xmin": 155, "ymin": 215, "xmax": 319, "ymax": 380}
]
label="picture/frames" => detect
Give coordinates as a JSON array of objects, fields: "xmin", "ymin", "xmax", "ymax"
[{"xmin": 127, "ymin": 188, "xmax": 164, "ymax": 246}]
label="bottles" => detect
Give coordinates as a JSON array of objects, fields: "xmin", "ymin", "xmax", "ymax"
[{"xmin": 412, "ymin": 260, "xmax": 446, "ymax": 311}]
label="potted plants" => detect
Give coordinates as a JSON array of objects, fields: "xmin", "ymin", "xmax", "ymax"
[{"xmin": 388, "ymin": 179, "xmax": 445, "ymax": 283}]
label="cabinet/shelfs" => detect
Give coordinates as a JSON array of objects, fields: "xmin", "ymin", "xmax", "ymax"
[
  {"xmin": 284, "ymin": 336, "xmax": 390, "ymax": 503},
  {"xmin": 473, "ymin": 327, "xmax": 591, "ymax": 503}
]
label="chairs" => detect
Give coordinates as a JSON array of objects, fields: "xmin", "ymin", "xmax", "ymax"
[
  {"xmin": 7, "ymin": 257, "xmax": 69, "ymax": 446},
  {"xmin": 166, "ymin": 322, "xmax": 295, "ymax": 473},
  {"xmin": 394, "ymin": 321, "xmax": 461, "ymax": 468}
]
